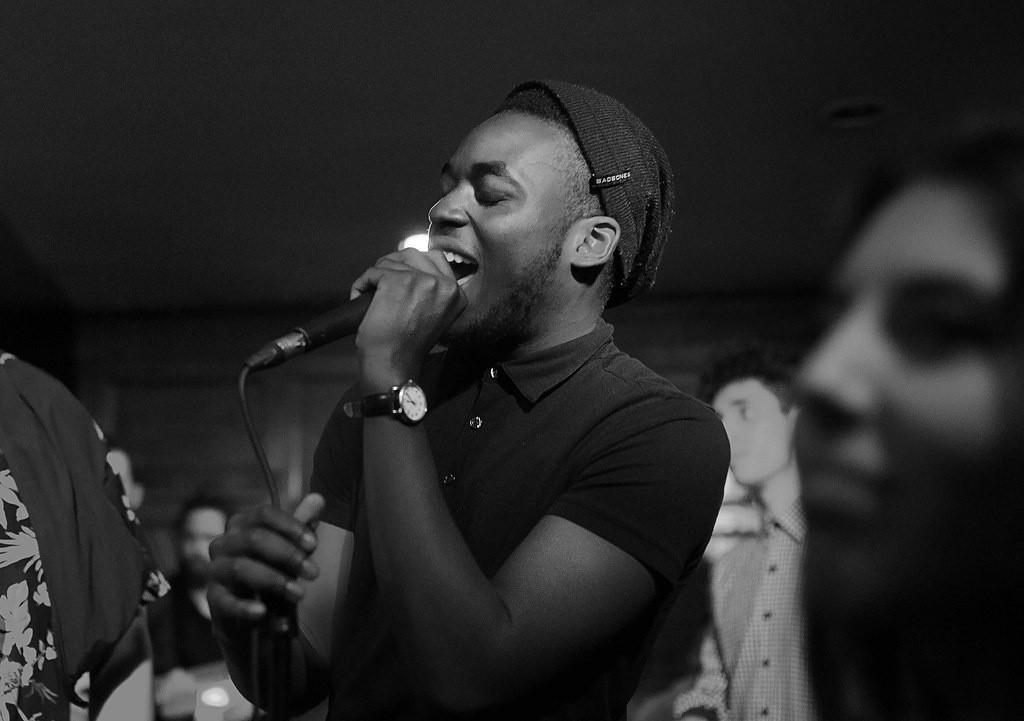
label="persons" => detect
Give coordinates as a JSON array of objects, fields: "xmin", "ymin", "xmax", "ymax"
[
  {"xmin": 0, "ymin": 351, "xmax": 150, "ymax": 721},
  {"xmin": 104, "ymin": 440, "xmax": 150, "ymax": 517},
  {"xmin": 148, "ymin": 498, "xmax": 258, "ymax": 721},
  {"xmin": 629, "ymin": 355, "xmax": 824, "ymax": 721},
  {"xmin": 793, "ymin": 142, "xmax": 1024, "ymax": 721},
  {"xmin": 207, "ymin": 76, "xmax": 732, "ymax": 721}
]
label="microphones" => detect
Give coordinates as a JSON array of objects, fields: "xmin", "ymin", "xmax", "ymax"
[{"xmin": 243, "ymin": 287, "xmax": 376, "ymax": 374}]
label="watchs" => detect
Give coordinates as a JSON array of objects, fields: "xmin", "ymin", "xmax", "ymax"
[{"xmin": 343, "ymin": 381, "xmax": 430, "ymax": 425}]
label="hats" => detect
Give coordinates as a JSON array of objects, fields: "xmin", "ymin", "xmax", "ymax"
[{"xmin": 504, "ymin": 80, "xmax": 676, "ymax": 311}]
